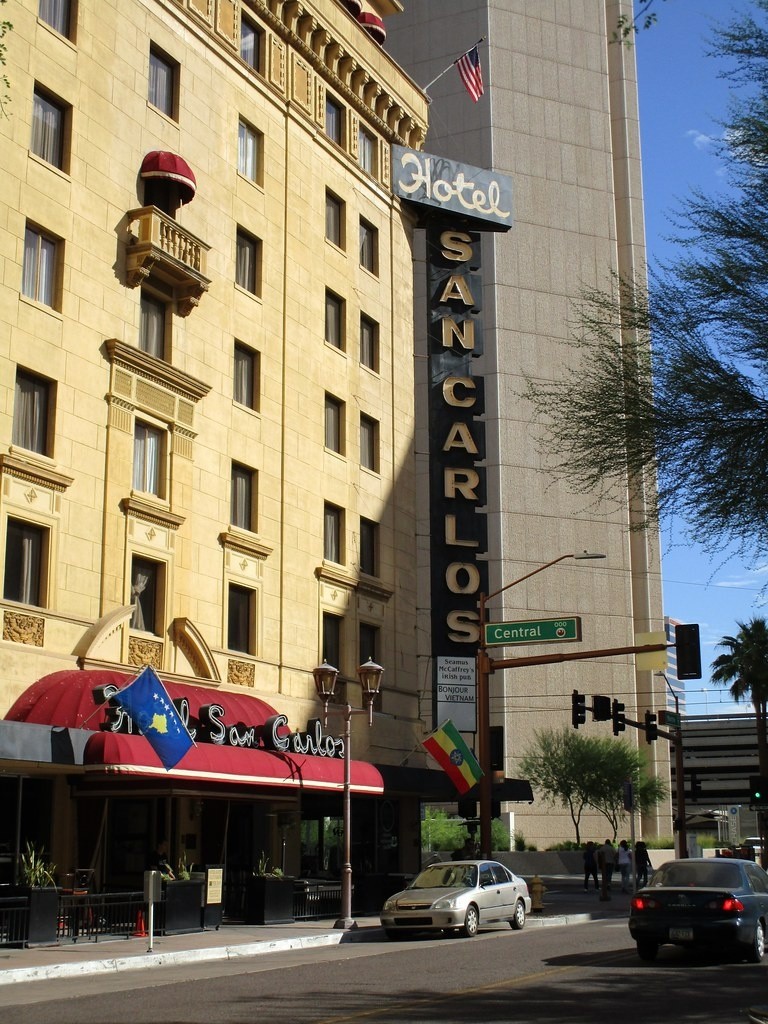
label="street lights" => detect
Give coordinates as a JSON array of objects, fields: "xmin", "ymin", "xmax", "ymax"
[
  {"xmin": 480, "ymin": 554, "xmax": 607, "ymax": 860},
  {"xmin": 654, "ymin": 671, "xmax": 688, "ymax": 859},
  {"xmin": 313, "ymin": 656, "xmax": 385, "ymax": 931}
]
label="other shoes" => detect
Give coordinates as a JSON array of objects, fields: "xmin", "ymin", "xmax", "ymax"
[{"xmin": 584, "ymin": 881, "xmax": 648, "ymax": 893}]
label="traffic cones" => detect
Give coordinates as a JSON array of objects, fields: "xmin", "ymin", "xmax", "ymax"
[
  {"xmin": 530, "ymin": 874, "xmax": 547, "ymax": 913},
  {"xmin": 83, "ymin": 908, "xmax": 93, "ymax": 929},
  {"xmin": 132, "ymin": 910, "xmax": 148, "ymax": 937}
]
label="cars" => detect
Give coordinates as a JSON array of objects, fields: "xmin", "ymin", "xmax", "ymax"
[
  {"xmin": 629, "ymin": 858, "xmax": 768, "ymax": 963},
  {"xmin": 743, "ymin": 837, "xmax": 764, "ymax": 854},
  {"xmin": 379, "ymin": 860, "xmax": 531, "ymax": 939}
]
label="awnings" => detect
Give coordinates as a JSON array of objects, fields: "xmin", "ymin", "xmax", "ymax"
[
  {"xmin": 83, "ymin": 733, "xmax": 384, "ymax": 794},
  {"xmin": 338, "ymin": 0, "xmax": 386, "ymax": 45},
  {"xmin": 140, "ymin": 151, "xmax": 198, "ymax": 206}
]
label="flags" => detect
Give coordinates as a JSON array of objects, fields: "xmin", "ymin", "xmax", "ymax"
[
  {"xmin": 110, "ymin": 665, "xmax": 193, "ymax": 772},
  {"xmin": 453, "ymin": 44, "xmax": 484, "ymax": 103},
  {"xmin": 422, "ymin": 719, "xmax": 484, "ymax": 795}
]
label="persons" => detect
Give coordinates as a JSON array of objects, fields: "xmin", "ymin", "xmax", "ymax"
[
  {"xmin": 421, "ymin": 838, "xmax": 482, "ymax": 871},
  {"xmin": 300, "ymin": 843, "xmax": 344, "ymax": 880},
  {"xmin": 582, "ymin": 839, "xmax": 653, "ymax": 894}
]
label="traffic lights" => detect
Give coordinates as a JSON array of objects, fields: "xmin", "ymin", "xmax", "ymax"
[
  {"xmin": 645, "ymin": 710, "xmax": 657, "ymax": 745},
  {"xmin": 612, "ymin": 699, "xmax": 626, "ymax": 736},
  {"xmin": 749, "ymin": 776, "xmax": 762, "ymax": 805},
  {"xmin": 572, "ymin": 690, "xmax": 586, "ymax": 729}
]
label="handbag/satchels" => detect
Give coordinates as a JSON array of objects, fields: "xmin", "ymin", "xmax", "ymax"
[
  {"xmin": 646, "ymin": 865, "xmax": 653, "ymax": 873},
  {"xmin": 614, "ymin": 847, "xmax": 620, "ymax": 859},
  {"xmin": 451, "ymin": 846, "xmax": 467, "ymax": 861}
]
